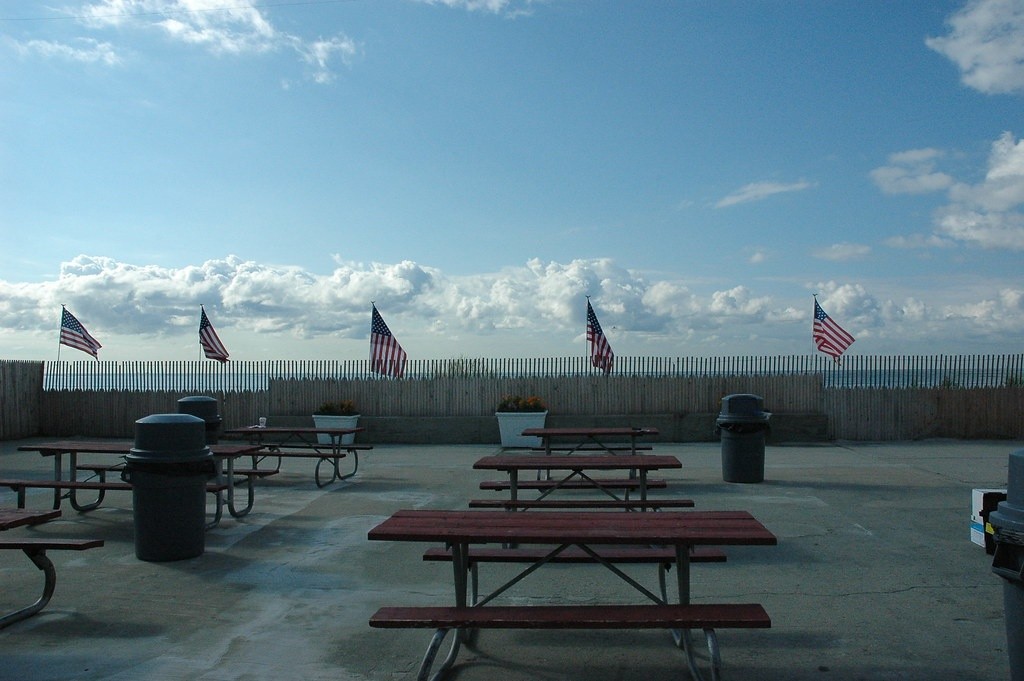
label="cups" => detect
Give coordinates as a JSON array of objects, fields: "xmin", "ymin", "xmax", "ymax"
[{"xmin": 259, "ymin": 417, "xmax": 266, "ymax": 426}]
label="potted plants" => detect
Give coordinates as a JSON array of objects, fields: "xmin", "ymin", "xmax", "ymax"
[{"xmin": 312, "ymin": 400, "xmax": 361, "ymax": 445}]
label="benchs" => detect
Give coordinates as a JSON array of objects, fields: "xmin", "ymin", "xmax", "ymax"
[
  {"xmin": 0, "ymin": 445, "xmax": 375, "ymax": 532},
  {"xmin": 369, "ymin": 446, "xmax": 772, "ymax": 680},
  {"xmin": 0, "ymin": 537, "xmax": 104, "ymax": 628}
]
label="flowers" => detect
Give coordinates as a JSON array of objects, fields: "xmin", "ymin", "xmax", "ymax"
[{"xmin": 496, "ymin": 393, "xmax": 548, "ymax": 413}]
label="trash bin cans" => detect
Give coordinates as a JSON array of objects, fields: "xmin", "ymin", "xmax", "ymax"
[
  {"xmin": 125, "ymin": 413, "xmax": 214, "ymax": 562},
  {"xmin": 176, "ymin": 396, "xmax": 222, "ymax": 481},
  {"xmin": 988, "ymin": 448, "xmax": 1024, "ymax": 681},
  {"xmin": 717, "ymin": 393, "xmax": 769, "ymax": 483}
]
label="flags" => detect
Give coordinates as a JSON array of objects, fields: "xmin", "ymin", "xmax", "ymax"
[
  {"xmin": 369, "ymin": 303, "xmax": 407, "ymax": 378},
  {"xmin": 60, "ymin": 306, "xmax": 102, "ymax": 363},
  {"xmin": 199, "ymin": 306, "xmax": 229, "ymax": 363},
  {"xmin": 813, "ymin": 298, "xmax": 856, "ymax": 366},
  {"xmin": 587, "ymin": 299, "xmax": 614, "ymax": 376}
]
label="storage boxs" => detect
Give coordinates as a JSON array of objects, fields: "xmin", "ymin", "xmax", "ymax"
[{"xmin": 969, "ymin": 489, "xmax": 1007, "ymax": 548}]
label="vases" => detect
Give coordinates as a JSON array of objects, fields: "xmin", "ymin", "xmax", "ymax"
[{"xmin": 495, "ymin": 409, "xmax": 548, "ymax": 449}]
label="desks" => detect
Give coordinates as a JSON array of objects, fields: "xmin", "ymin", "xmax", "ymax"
[
  {"xmin": 470, "ymin": 453, "xmax": 681, "ymax": 550},
  {"xmin": 367, "ymin": 508, "xmax": 777, "ymax": 677},
  {"xmin": 225, "ymin": 428, "xmax": 365, "ymax": 486},
  {"xmin": 523, "ymin": 428, "xmax": 660, "ymax": 493},
  {"xmin": 17, "ymin": 442, "xmax": 265, "ymax": 530},
  {"xmin": 0, "ymin": 507, "xmax": 62, "ymax": 530}
]
